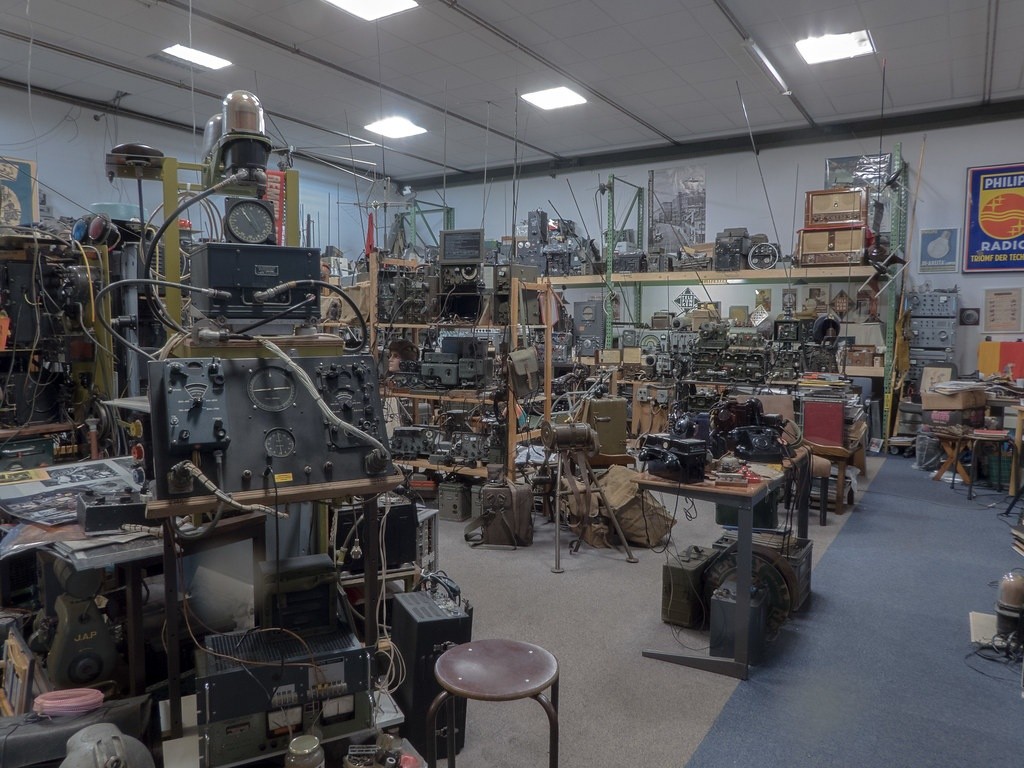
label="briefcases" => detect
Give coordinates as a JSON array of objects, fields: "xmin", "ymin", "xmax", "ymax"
[{"xmin": 661, "ymin": 544, "xmax": 719, "ymax": 627}]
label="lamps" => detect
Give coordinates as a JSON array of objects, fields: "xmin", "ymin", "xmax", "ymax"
[{"xmin": 742, "ymin": 38, "xmax": 792, "ymax": 96}]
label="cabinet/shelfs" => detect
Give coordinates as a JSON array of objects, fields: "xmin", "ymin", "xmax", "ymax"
[{"xmin": 319, "ymin": 251, "xmax": 904, "ymax": 518}]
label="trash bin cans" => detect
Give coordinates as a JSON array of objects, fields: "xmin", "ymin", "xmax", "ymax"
[{"xmin": 916, "ymin": 434, "xmax": 942, "ymax": 470}]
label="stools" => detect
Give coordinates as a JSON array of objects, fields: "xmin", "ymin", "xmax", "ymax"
[
  {"xmin": 950, "ymin": 434, "xmax": 1021, "ymax": 501},
  {"xmin": 426, "ymin": 638, "xmax": 560, "ymax": 768}
]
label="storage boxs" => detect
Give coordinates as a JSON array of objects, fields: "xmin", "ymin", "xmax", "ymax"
[{"xmin": 921, "ymin": 389, "xmax": 987, "ymax": 429}]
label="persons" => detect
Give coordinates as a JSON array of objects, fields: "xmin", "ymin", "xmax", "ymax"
[{"xmin": 388, "ymin": 340, "xmax": 420, "ymax": 372}]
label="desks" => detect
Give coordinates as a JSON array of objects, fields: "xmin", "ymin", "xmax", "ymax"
[{"xmin": 629, "ymin": 443, "xmax": 814, "ymax": 680}]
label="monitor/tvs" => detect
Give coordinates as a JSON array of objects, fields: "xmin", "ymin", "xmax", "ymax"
[{"xmin": 439, "ymin": 229, "xmax": 485, "ymax": 265}]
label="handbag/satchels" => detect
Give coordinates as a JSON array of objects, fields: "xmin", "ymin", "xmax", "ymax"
[{"xmin": 507, "ymin": 347, "xmax": 538, "ymax": 398}]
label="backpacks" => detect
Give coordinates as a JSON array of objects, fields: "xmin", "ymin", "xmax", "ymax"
[{"xmin": 558, "ymin": 464, "xmax": 678, "ymax": 548}]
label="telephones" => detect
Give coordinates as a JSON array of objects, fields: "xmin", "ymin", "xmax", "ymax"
[{"xmin": 728, "ymin": 424, "xmax": 784, "ymax": 464}]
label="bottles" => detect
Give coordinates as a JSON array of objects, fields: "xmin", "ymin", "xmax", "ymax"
[{"xmin": 285, "ymin": 735, "xmax": 325, "ymax": 768}]
